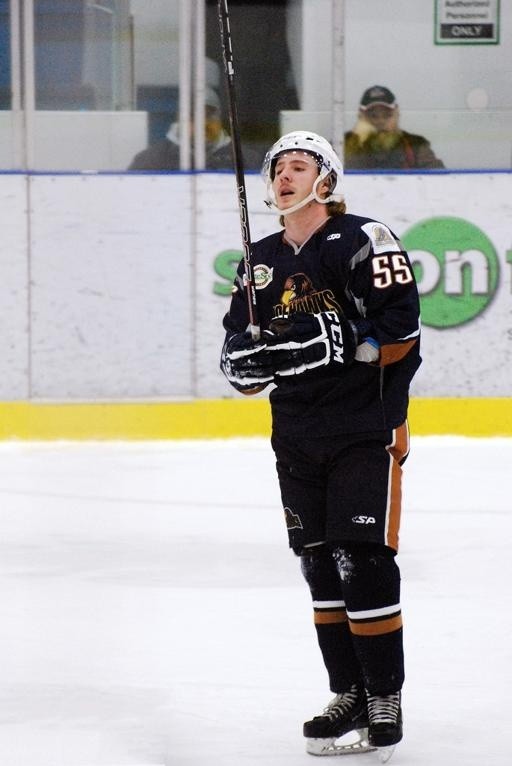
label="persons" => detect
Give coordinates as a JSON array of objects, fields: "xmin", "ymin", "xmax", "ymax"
[
  {"xmin": 218, "ymin": 128, "xmax": 422, "ymax": 748},
  {"xmin": 327, "ymin": 85, "xmax": 448, "ymax": 171},
  {"xmin": 126, "ymin": 84, "xmax": 262, "ymax": 170}
]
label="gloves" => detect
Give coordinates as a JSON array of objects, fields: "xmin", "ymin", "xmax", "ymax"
[
  {"xmin": 257, "ymin": 307, "xmax": 358, "ymax": 384},
  {"xmin": 217, "ymin": 331, "xmax": 275, "ymax": 391}
]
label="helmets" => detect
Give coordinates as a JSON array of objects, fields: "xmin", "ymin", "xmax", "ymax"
[{"xmin": 260, "ymin": 130, "xmax": 347, "ymax": 216}]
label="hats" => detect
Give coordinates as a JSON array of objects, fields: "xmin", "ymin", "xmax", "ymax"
[
  {"xmin": 357, "ymin": 85, "xmax": 398, "ymax": 111},
  {"xmin": 206, "ymin": 88, "xmax": 223, "ymax": 111}
]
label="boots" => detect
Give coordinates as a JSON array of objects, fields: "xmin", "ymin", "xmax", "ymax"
[
  {"xmin": 301, "ymin": 682, "xmax": 369, "ymax": 739},
  {"xmin": 363, "ymin": 686, "xmax": 404, "ymax": 748}
]
